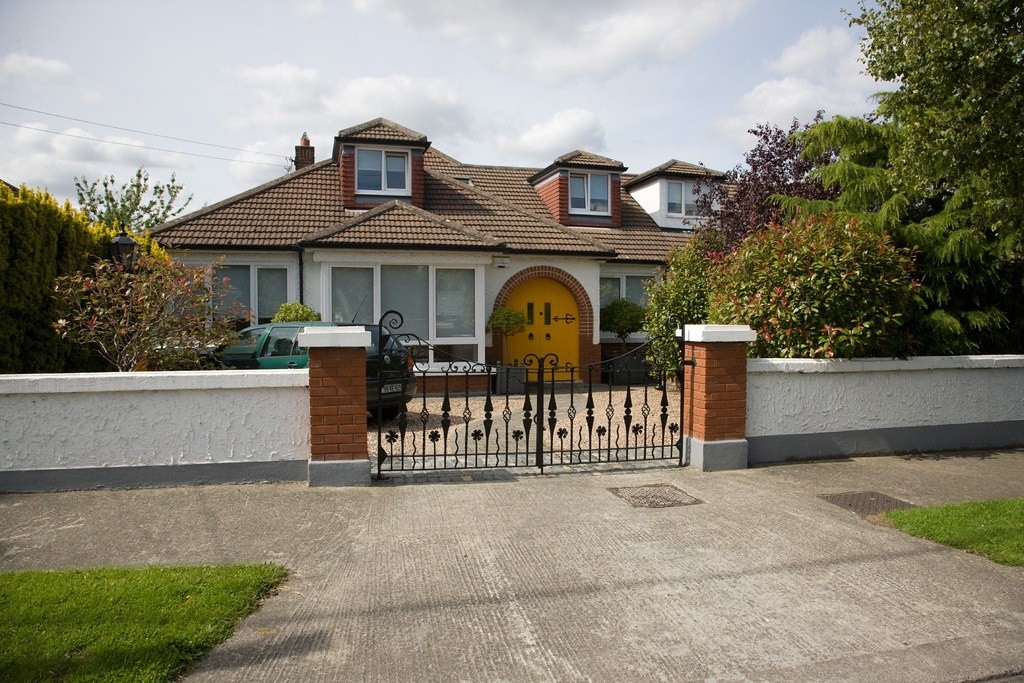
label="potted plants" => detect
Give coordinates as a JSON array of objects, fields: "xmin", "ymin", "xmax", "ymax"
[
  {"xmin": 600, "ymin": 298, "xmax": 648, "ymax": 385},
  {"xmin": 485, "ymin": 306, "xmax": 531, "ymax": 394}
]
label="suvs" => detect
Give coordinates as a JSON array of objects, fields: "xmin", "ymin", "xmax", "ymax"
[{"xmin": 151, "ymin": 319, "xmax": 418, "ymax": 421}]
